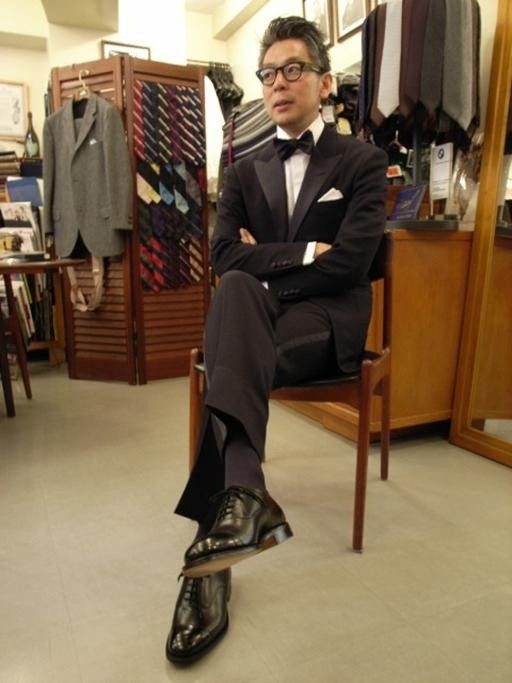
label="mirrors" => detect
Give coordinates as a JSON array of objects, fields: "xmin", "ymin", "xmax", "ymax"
[{"xmin": 447, "ymin": 0, "xmax": 511, "ymax": 470}]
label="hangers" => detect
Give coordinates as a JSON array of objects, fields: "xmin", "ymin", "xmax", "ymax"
[
  {"xmin": 207, "ymin": 60, "xmax": 244, "ymax": 102},
  {"xmin": 72, "ymin": 69, "xmax": 91, "ymax": 106}
]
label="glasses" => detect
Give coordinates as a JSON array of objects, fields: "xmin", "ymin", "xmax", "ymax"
[{"xmin": 256, "ymin": 62, "xmax": 327, "ymax": 85}]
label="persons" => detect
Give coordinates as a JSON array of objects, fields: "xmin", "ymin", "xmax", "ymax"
[{"xmin": 166, "ymin": 16, "xmax": 388, "ymax": 666}]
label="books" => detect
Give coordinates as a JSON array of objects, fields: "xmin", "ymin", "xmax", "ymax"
[{"xmin": 0, "ymin": 150, "xmax": 47, "ymax": 351}]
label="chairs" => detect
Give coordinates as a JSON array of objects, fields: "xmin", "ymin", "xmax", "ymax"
[{"xmin": 188, "ymin": 228, "xmax": 395, "ymax": 553}]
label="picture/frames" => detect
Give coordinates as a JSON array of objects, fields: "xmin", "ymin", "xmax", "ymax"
[
  {"xmin": 0, "ymin": 78, "xmax": 30, "ymax": 142},
  {"xmin": 302, "ymin": 0, "xmax": 371, "ymax": 51}
]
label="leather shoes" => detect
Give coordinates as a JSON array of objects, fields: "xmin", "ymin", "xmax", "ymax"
[
  {"xmin": 183, "ymin": 485, "xmax": 295, "ymax": 577},
  {"xmin": 165, "ymin": 568, "xmax": 232, "ymax": 666}
]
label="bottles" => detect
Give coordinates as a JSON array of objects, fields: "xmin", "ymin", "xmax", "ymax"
[{"xmin": 22, "ymin": 109, "xmax": 41, "ymax": 159}]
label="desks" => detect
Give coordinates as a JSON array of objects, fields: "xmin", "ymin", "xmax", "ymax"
[
  {"xmin": 0, "ymin": 258, "xmax": 86, "ymax": 377},
  {"xmin": 276, "ymin": 228, "xmax": 471, "ymax": 444}
]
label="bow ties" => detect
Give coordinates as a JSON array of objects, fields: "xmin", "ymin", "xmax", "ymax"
[{"xmin": 272, "ymin": 130, "xmax": 314, "ymax": 161}]
